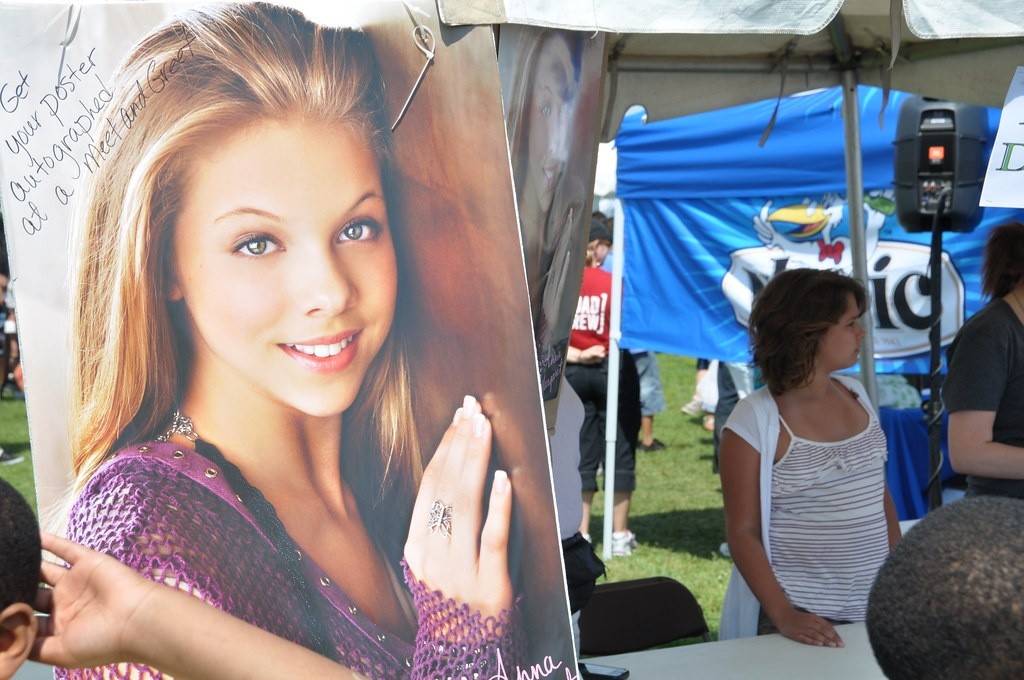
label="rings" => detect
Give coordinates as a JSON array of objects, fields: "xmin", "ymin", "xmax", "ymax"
[{"xmin": 427, "ymin": 501, "xmax": 452, "ymax": 536}]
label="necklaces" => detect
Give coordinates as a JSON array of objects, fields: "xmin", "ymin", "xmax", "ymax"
[{"xmin": 156, "ymin": 410, "xmax": 335, "ymax": 658}]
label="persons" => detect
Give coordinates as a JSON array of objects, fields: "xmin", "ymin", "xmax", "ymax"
[
  {"xmin": 57, "ymin": 2, "xmax": 530, "ymax": 680},
  {"xmin": 0, "ymin": 477, "xmax": 370, "ymax": 680},
  {"xmin": 562, "ymin": 211, "xmax": 641, "ymax": 556},
  {"xmin": 630, "ymin": 350, "xmax": 751, "ymax": 558},
  {"xmin": 944, "ymin": 225, "xmax": 1023, "ymax": 499},
  {"xmin": 867, "ymin": 497, "xmax": 1023, "ymax": 680},
  {"xmin": 719, "ymin": 269, "xmax": 903, "ymax": 650}
]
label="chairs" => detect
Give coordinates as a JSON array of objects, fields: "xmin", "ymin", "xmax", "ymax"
[{"xmin": 578, "ymin": 577, "xmax": 711, "ymax": 659}]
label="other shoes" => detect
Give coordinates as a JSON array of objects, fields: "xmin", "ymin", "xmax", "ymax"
[
  {"xmin": 703, "ymin": 414, "xmax": 715, "ymax": 431},
  {"xmin": 612, "ymin": 529, "xmax": 635, "ymax": 556},
  {"xmin": 681, "ymin": 400, "xmax": 702, "ymax": 416},
  {"xmin": 641, "ymin": 438, "xmax": 665, "ymax": 451}
]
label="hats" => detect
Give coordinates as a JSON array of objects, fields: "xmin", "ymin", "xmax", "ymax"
[{"xmin": 589, "ymin": 218, "xmax": 612, "ymax": 240}]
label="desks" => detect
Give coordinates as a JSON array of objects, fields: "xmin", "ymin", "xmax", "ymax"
[{"xmin": 580, "ymin": 621, "xmax": 889, "ymax": 680}]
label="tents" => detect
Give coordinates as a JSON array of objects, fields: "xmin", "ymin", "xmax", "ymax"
[
  {"xmin": 602, "ymin": 80, "xmax": 1024, "ymax": 565},
  {"xmin": 503, "ymin": 0, "xmax": 1024, "ymax": 419}
]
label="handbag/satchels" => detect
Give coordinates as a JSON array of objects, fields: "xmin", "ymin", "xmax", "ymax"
[{"xmin": 561, "ymin": 531, "xmax": 607, "ymax": 615}]
